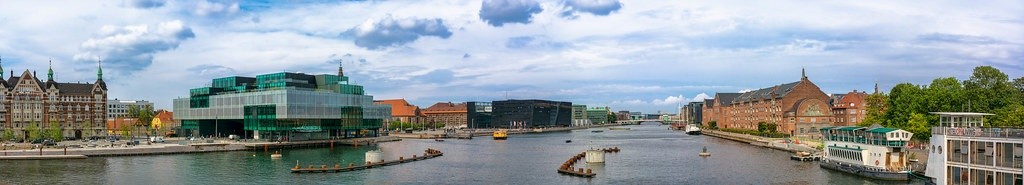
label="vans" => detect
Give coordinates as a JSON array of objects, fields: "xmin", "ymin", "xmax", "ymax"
[{"xmin": 229, "ymin": 135, "xmax": 240, "ymax": 140}]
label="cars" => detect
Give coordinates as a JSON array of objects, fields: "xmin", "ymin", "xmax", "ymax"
[
  {"xmin": 31, "ymin": 139, "xmax": 58, "ymax": 146},
  {"xmin": 151, "ymin": 136, "xmax": 165, "ymax": 143}
]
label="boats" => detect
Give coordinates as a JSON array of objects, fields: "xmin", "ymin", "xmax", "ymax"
[
  {"xmin": 685, "ymin": 124, "xmax": 701, "ymax": 135},
  {"xmin": 493, "ymin": 129, "xmax": 508, "ymax": 140},
  {"xmin": 819, "ymin": 126, "xmax": 915, "ymax": 181}
]
